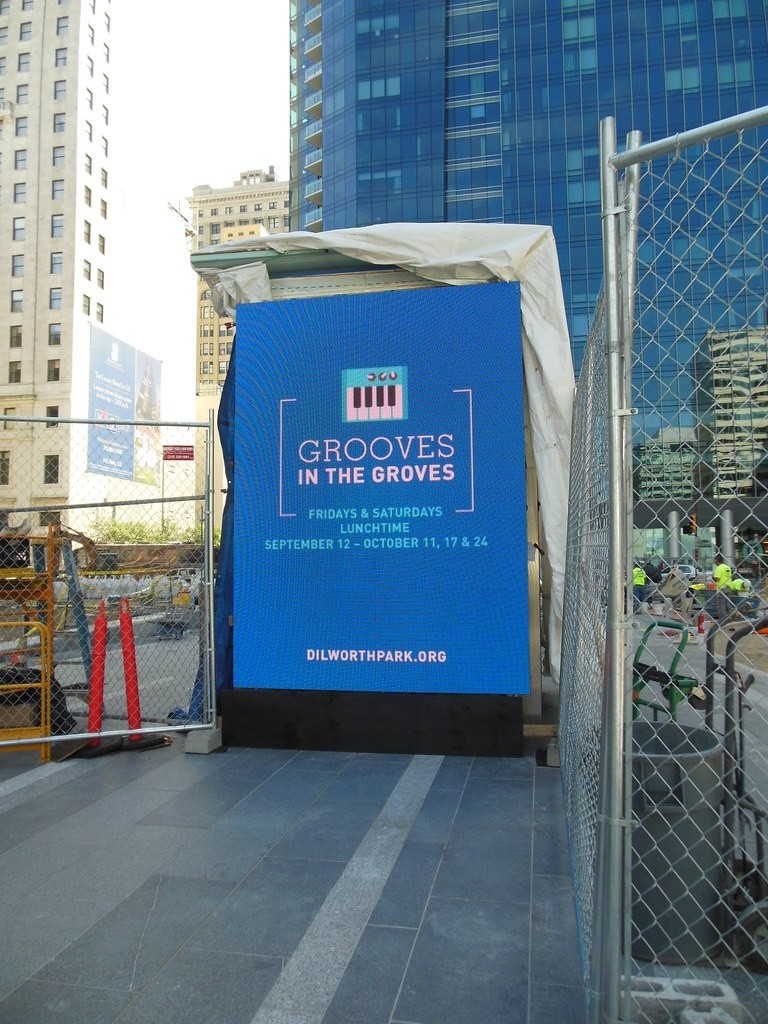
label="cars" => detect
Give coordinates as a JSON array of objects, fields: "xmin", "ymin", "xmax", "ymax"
[{"xmin": 661, "ymin": 565, "xmax": 696, "ymax": 581}]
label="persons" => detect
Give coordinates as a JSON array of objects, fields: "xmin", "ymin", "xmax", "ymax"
[
  {"xmin": 632, "ymin": 562, "xmax": 647, "ymax": 614},
  {"xmin": 150, "ymin": 587, "xmax": 192, "ymax": 637},
  {"xmin": 711, "ymin": 553, "xmax": 732, "ymax": 618},
  {"xmin": 731, "ymin": 579, "xmax": 751, "ymax": 595}
]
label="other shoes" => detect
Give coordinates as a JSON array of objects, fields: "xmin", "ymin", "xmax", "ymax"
[
  {"xmin": 717, "ymin": 615, "xmax": 730, "ymax": 623},
  {"xmin": 634, "ymin": 609, "xmax": 640, "ymax": 614}
]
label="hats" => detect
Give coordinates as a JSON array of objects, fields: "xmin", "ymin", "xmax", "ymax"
[{"xmin": 743, "ymin": 579, "xmax": 751, "ymax": 587}]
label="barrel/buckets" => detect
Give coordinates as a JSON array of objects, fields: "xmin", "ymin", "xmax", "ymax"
[{"xmin": 53, "ymin": 574, "xmax": 191, "ymax": 607}]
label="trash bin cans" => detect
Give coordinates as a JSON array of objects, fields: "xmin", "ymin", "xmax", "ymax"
[{"xmin": 624, "ymin": 719, "xmax": 728, "ymax": 966}]
